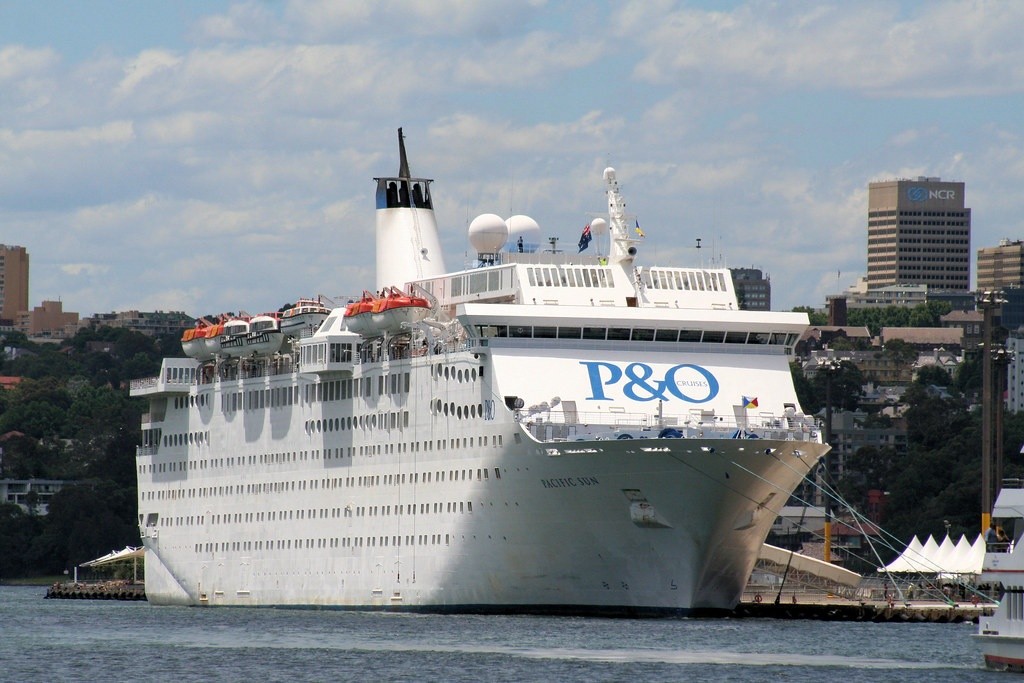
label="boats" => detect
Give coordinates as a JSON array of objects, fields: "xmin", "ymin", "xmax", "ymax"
[
  {"xmin": 977, "ymin": 482, "xmax": 1023, "ymax": 663},
  {"xmin": 180, "ymin": 281, "xmax": 443, "ymax": 362}
]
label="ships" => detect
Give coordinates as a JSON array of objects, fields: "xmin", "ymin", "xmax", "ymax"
[{"xmin": 129, "ymin": 128, "xmax": 832, "ymax": 615}]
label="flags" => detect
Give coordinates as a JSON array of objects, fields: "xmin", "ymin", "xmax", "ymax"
[
  {"xmin": 744, "ymin": 397, "xmax": 758, "ymax": 409},
  {"xmin": 635, "ymin": 220, "xmax": 644, "ymax": 237},
  {"xmin": 578, "ymin": 225, "xmax": 592, "ymax": 254}
]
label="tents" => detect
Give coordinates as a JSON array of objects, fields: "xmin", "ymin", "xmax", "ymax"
[{"xmin": 877, "ymin": 535, "xmax": 987, "ymax": 574}]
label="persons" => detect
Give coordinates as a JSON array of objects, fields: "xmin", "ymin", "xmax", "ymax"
[
  {"xmin": 884, "ymin": 582, "xmax": 1004, "ymax": 601},
  {"xmin": 598, "ymin": 257, "xmax": 606, "ymax": 265},
  {"xmin": 985, "ymin": 523, "xmax": 1009, "ymax": 552},
  {"xmin": 517, "ymin": 236, "xmax": 523, "ymax": 253}
]
